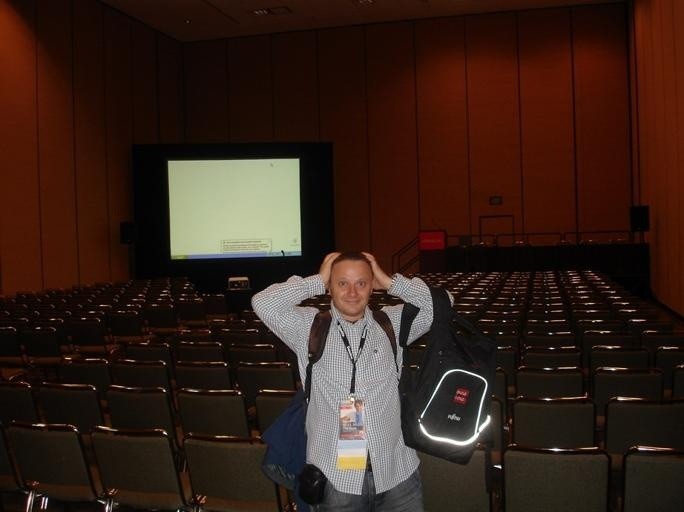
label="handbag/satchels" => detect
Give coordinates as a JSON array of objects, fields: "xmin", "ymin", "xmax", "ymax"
[{"xmin": 262, "ymin": 389, "xmax": 307, "ymax": 490}]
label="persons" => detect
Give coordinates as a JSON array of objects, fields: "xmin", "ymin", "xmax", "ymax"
[
  {"xmin": 251, "ymin": 249, "xmax": 455, "ymax": 511},
  {"xmin": 354, "ymin": 400, "xmax": 363, "ymax": 432}
]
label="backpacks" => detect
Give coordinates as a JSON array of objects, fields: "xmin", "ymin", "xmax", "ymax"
[{"xmin": 398, "ymin": 284, "xmax": 496, "ymax": 465}]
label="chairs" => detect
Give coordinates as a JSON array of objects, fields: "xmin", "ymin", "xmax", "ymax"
[{"xmin": 0, "ymin": 261, "xmax": 683, "ymax": 512}]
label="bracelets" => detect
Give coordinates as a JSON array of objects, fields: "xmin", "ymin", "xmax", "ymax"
[{"xmin": 388, "ymin": 275, "xmax": 396, "ymax": 292}]
